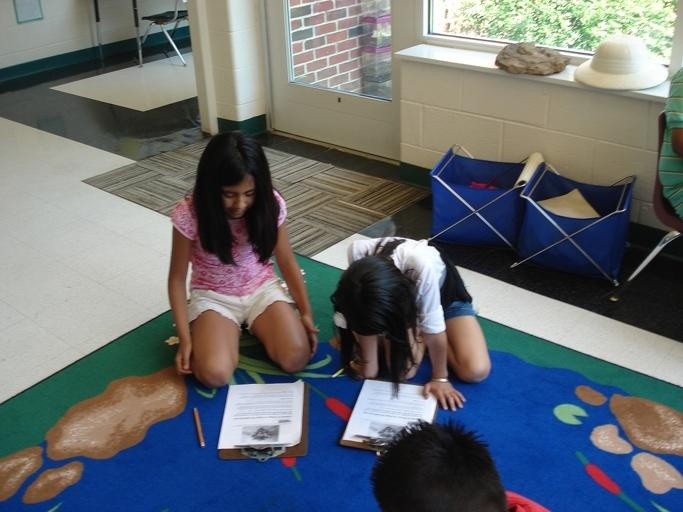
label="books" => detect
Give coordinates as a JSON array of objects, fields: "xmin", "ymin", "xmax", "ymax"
[
  {"xmin": 342, "ymin": 379, "xmax": 437, "ymax": 446},
  {"xmin": 217, "ymin": 379, "xmax": 304, "ymax": 449}
]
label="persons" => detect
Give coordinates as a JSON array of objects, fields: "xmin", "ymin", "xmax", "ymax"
[
  {"xmin": 168, "ymin": 129, "xmax": 320, "ymax": 388},
  {"xmin": 331, "ymin": 236, "xmax": 491, "ymax": 411},
  {"xmin": 659, "ymin": 67, "xmax": 683, "ymax": 221},
  {"xmin": 369, "ymin": 419, "xmax": 550, "ymax": 512}
]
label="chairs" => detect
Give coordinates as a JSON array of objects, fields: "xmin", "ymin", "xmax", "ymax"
[{"xmin": 139, "ymin": 1, "xmax": 188, "ymax": 68}]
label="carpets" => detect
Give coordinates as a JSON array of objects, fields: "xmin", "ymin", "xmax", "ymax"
[
  {"xmin": 361, "ymin": 186, "xmax": 683, "ymax": 341},
  {"xmin": 82, "ymin": 137, "xmax": 430, "ymax": 258},
  {"xmin": 0, "ymin": 250, "xmax": 683, "ymax": 511}
]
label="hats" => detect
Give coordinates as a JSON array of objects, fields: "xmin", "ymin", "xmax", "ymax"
[{"xmin": 573, "ymin": 33, "xmax": 670, "ymax": 93}]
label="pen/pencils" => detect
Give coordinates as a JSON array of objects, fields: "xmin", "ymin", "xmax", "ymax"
[
  {"xmin": 332, "ymin": 357, "xmax": 357, "ymax": 379},
  {"xmin": 193, "ymin": 407, "xmax": 206, "ymax": 448}
]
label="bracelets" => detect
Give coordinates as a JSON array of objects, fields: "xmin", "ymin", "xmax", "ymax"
[{"xmin": 431, "ymin": 377, "xmax": 449, "ymax": 382}]
